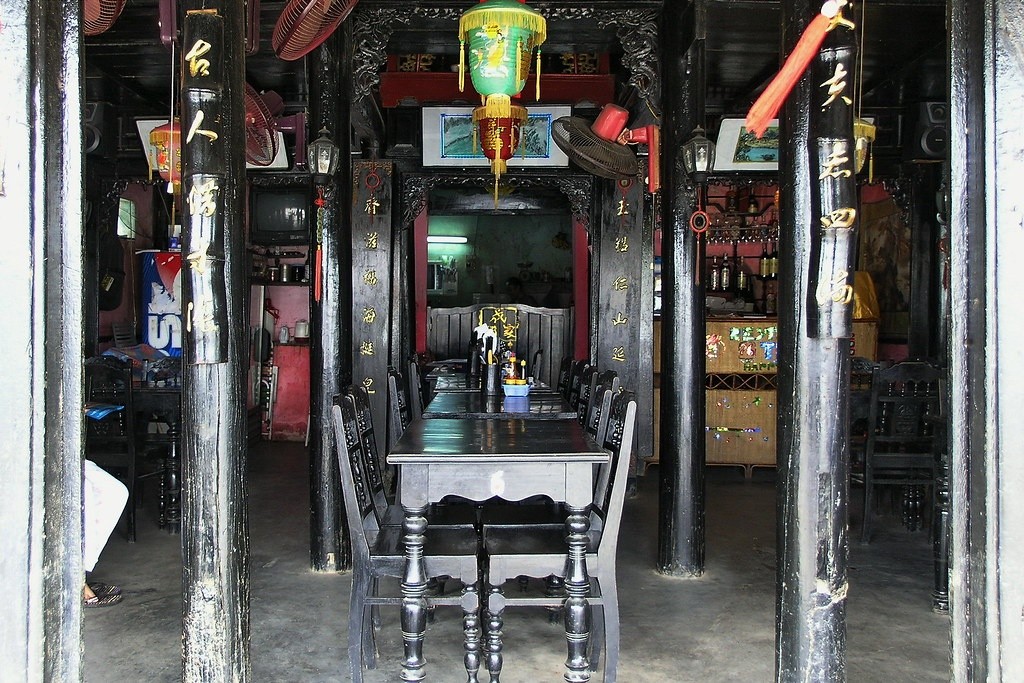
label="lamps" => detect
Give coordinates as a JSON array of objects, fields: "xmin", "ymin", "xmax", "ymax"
[
  {"xmin": 306, "ymin": 126, "xmax": 340, "ymax": 303},
  {"xmin": 680, "ymin": 124, "xmax": 716, "ymax": 285}
]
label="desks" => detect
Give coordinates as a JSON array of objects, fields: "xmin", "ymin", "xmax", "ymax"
[
  {"xmin": 425, "ymin": 367, "xmax": 467, "ymax": 403},
  {"xmin": 432, "ymin": 376, "xmax": 553, "ymax": 394},
  {"xmin": 385, "ymin": 416, "xmax": 609, "ymax": 683},
  {"xmin": 422, "ymin": 392, "xmax": 578, "ymax": 420},
  {"xmin": 425, "ymin": 358, "xmax": 468, "ymax": 368},
  {"xmin": 92, "ymin": 378, "xmax": 181, "ymax": 535}
]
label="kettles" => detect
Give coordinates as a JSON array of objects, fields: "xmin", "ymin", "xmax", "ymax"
[{"xmin": 294, "ymin": 320, "xmax": 309, "ymax": 344}]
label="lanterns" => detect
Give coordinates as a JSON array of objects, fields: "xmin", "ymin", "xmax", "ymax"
[
  {"xmin": 854, "ymin": 116, "xmax": 878, "ymax": 184},
  {"xmin": 149, "ymin": 116, "xmax": 181, "ymax": 225},
  {"xmin": 459, "ymin": 0, "xmax": 547, "ymax": 118},
  {"xmin": 472, "ymin": 104, "xmax": 528, "ymax": 208}
]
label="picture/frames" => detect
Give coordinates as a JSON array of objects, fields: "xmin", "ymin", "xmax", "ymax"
[
  {"xmin": 711, "ymin": 114, "xmax": 875, "ymax": 174},
  {"xmin": 419, "ymin": 103, "xmax": 574, "ymax": 169}
]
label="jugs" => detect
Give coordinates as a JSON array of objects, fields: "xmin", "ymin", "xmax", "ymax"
[{"xmin": 264, "ymin": 298, "xmax": 279, "ymax": 341}]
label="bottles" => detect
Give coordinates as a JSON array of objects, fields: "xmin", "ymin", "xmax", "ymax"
[
  {"xmin": 502, "ymin": 341, "xmax": 528, "ymax": 384},
  {"xmin": 736, "ymin": 256, "xmax": 748, "ymax": 292},
  {"xmin": 708, "ymin": 184, "xmax": 778, "ymax": 240},
  {"xmin": 709, "ymin": 252, "xmax": 730, "ymax": 293},
  {"xmin": 759, "ymin": 242, "xmax": 778, "ymax": 280}
]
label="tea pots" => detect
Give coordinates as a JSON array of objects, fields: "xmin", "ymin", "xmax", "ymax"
[{"xmin": 279, "ymin": 325, "xmax": 289, "ymax": 343}]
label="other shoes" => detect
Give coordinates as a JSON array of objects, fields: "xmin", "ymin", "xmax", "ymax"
[
  {"xmin": 83, "ymin": 594, "xmax": 121, "ymax": 607},
  {"xmin": 86, "ymin": 581, "xmax": 120, "ymax": 597}
]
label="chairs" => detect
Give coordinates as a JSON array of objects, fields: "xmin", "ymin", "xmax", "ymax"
[
  {"xmin": 82, "ymin": 355, "xmax": 168, "ymax": 544},
  {"xmin": 850, "ymin": 355, "xmax": 952, "ymax": 544},
  {"xmin": 332, "ymin": 350, "xmax": 636, "ymax": 683}
]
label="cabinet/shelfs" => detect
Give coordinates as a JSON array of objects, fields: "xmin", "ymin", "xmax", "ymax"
[
  {"xmin": 653, "ymin": 201, "xmax": 779, "ymax": 481},
  {"xmin": 260, "ymin": 345, "xmax": 309, "ymax": 442}
]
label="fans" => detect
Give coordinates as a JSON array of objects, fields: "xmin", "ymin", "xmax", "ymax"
[
  {"xmin": 243, "ymin": 81, "xmax": 308, "ymax": 172},
  {"xmin": 550, "ymin": 104, "xmax": 659, "ymax": 194},
  {"xmin": 244, "ymin": 0, "xmax": 359, "ymax": 61},
  {"xmin": 83, "ymin": 0, "xmax": 180, "ymax": 193}
]
